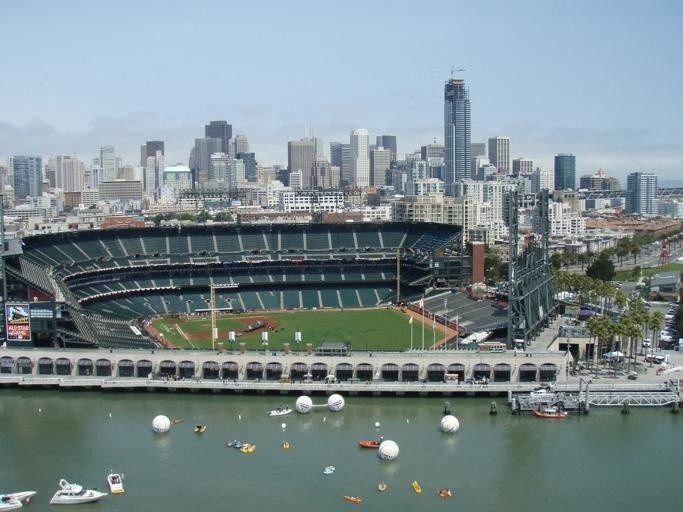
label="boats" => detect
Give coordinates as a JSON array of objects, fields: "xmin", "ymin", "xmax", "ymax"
[
  {"xmin": 532, "ymin": 406, "xmax": 568, "ymax": 418},
  {"xmin": 193, "ymin": 406, "xmax": 451, "ymax": 503},
  {"xmin": 0, "ymin": 474, "xmax": 125, "ymax": 511}
]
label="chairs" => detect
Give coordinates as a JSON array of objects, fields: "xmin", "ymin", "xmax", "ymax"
[
  {"xmin": 4, "ymin": 220, "xmax": 461, "ymax": 349},
  {"xmin": 407, "ymin": 286, "xmax": 509, "ymax": 346}
]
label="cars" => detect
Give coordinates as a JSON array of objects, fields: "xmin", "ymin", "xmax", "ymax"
[
  {"xmin": 659, "ymin": 301, "xmax": 680, "ymax": 350},
  {"xmin": 490, "ymin": 348, "xmax": 504, "ymax": 353},
  {"xmin": 643, "ymin": 339, "xmax": 651, "ymax": 347}
]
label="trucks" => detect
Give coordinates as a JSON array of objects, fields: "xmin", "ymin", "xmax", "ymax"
[{"xmin": 646, "ymin": 354, "xmax": 664, "ymax": 364}]
